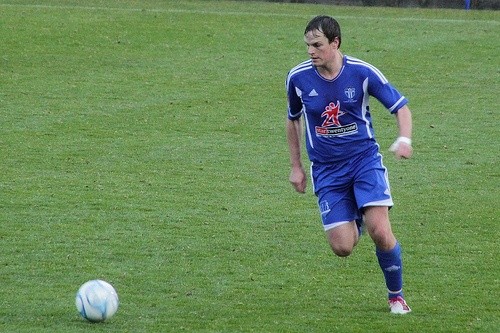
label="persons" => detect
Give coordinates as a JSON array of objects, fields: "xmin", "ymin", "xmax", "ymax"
[{"xmin": 285, "ymin": 15, "xmax": 412, "ymax": 313}]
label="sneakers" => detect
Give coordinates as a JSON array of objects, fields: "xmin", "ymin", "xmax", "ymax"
[{"xmin": 388, "ymin": 296, "xmax": 412, "ymax": 315}]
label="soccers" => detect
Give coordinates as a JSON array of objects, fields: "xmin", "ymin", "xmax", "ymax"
[{"xmin": 74, "ymin": 279, "xmax": 120, "ymax": 323}]
